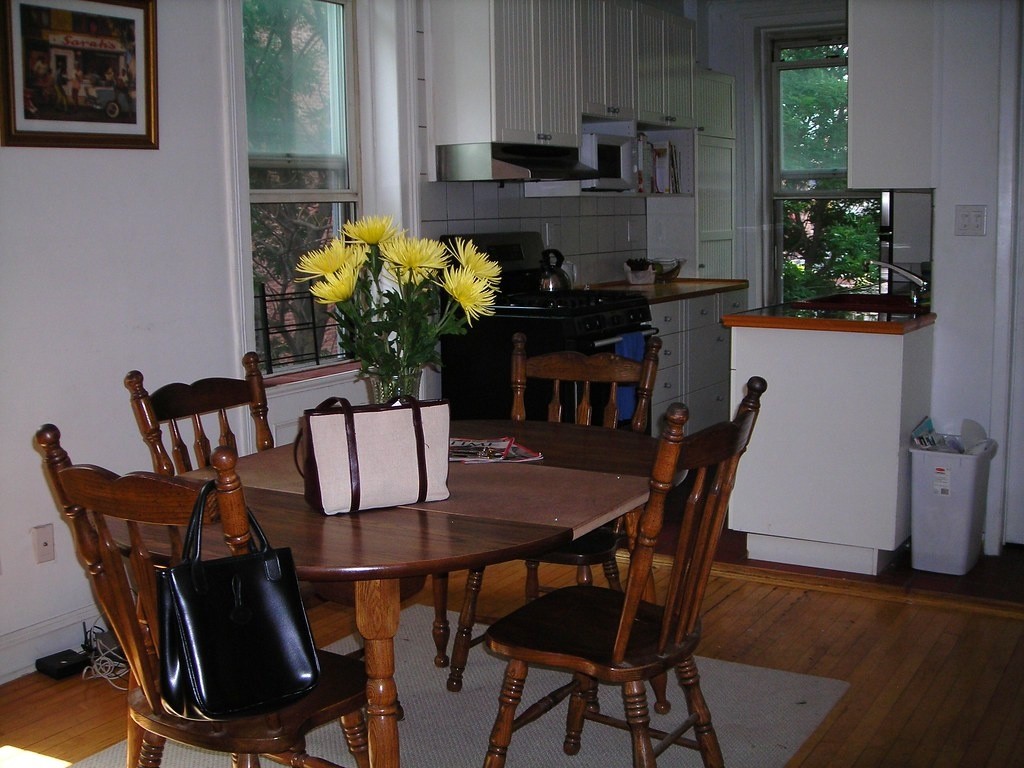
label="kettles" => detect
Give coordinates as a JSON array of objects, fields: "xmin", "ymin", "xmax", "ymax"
[{"xmin": 538, "ymin": 249, "xmax": 570, "ymax": 292}]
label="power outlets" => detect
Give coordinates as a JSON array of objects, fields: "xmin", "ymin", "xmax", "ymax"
[{"xmin": 956, "ymin": 205, "xmax": 985, "ymax": 236}]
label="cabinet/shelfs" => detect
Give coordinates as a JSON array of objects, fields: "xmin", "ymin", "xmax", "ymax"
[
  {"xmin": 729, "ymin": 325, "xmax": 935, "ymax": 576},
  {"xmin": 423, "ymin": 0, "xmax": 696, "ymax": 198},
  {"xmin": 848, "ymin": 0, "xmax": 940, "ymax": 188},
  {"xmin": 647, "ymin": 69, "xmax": 735, "ymax": 280},
  {"xmin": 589, "ymin": 277, "xmax": 749, "ymax": 438}
]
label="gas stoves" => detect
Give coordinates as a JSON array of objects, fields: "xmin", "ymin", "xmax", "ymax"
[{"xmin": 440, "ymin": 232, "xmax": 652, "ymax": 312}]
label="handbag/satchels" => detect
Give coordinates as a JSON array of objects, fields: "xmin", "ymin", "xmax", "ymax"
[
  {"xmin": 155, "ymin": 476, "xmax": 322, "ymax": 721},
  {"xmin": 294, "ymin": 395, "xmax": 451, "ymax": 516}
]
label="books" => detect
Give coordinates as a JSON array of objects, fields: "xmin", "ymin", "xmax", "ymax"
[
  {"xmin": 449, "ymin": 437, "xmax": 543, "ymax": 465},
  {"xmin": 637, "ymin": 133, "xmax": 680, "ymax": 193}
]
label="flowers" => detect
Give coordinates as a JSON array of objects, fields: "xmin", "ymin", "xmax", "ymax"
[{"xmin": 296, "ymin": 214, "xmax": 502, "ymax": 402}]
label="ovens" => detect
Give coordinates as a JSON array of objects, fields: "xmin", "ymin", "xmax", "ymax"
[{"xmin": 438, "ymin": 300, "xmax": 659, "ymax": 437}]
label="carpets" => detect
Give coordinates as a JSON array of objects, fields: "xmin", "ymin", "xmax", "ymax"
[{"xmin": 73, "ymin": 603, "xmax": 850, "ymax": 768}]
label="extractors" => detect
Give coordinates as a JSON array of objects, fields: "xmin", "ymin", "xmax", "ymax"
[{"xmin": 439, "ymin": 142, "xmax": 599, "ymax": 181}]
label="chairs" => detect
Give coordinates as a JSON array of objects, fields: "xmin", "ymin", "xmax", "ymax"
[{"xmin": 36, "ymin": 333, "xmax": 767, "ymax": 768}]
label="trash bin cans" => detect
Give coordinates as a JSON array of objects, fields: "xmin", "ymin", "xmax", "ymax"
[{"xmin": 907, "ymin": 433, "xmax": 991, "ymax": 577}]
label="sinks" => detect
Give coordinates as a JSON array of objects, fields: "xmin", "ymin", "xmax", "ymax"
[{"xmin": 791, "ymin": 294, "xmax": 930, "ymax": 314}]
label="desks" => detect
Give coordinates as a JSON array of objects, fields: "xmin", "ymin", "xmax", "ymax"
[{"xmin": 102, "ymin": 420, "xmax": 688, "ymax": 768}]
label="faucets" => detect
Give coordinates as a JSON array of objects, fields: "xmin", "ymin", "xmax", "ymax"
[{"xmin": 863, "ymin": 259, "xmax": 930, "ymax": 293}]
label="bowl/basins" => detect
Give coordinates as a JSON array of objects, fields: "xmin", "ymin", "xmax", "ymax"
[
  {"xmin": 627, "ymin": 271, "xmax": 652, "ymax": 284},
  {"xmin": 647, "ymin": 257, "xmax": 686, "ymax": 283}
]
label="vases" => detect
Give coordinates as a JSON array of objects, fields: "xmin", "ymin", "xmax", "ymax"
[{"xmin": 368, "ymin": 368, "xmax": 422, "ymax": 404}]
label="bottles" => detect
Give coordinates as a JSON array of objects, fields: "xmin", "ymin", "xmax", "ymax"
[
  {"xmin": 637, "ymin": 135, "xmax": 652, "ymax": 191},
  {"xmin": 911, "ymin": 284, "xmax": 920, "ymax": 304}
]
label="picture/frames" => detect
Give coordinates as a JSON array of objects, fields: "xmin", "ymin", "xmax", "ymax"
[{"xmin": 0, "ymin": 0, "xmax": 159, "ymax": 150}]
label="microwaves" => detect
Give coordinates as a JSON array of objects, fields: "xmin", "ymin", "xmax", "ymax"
[{"xmin": 582, "ymin": 131, "xmax": 632, "ymax": 192}]
